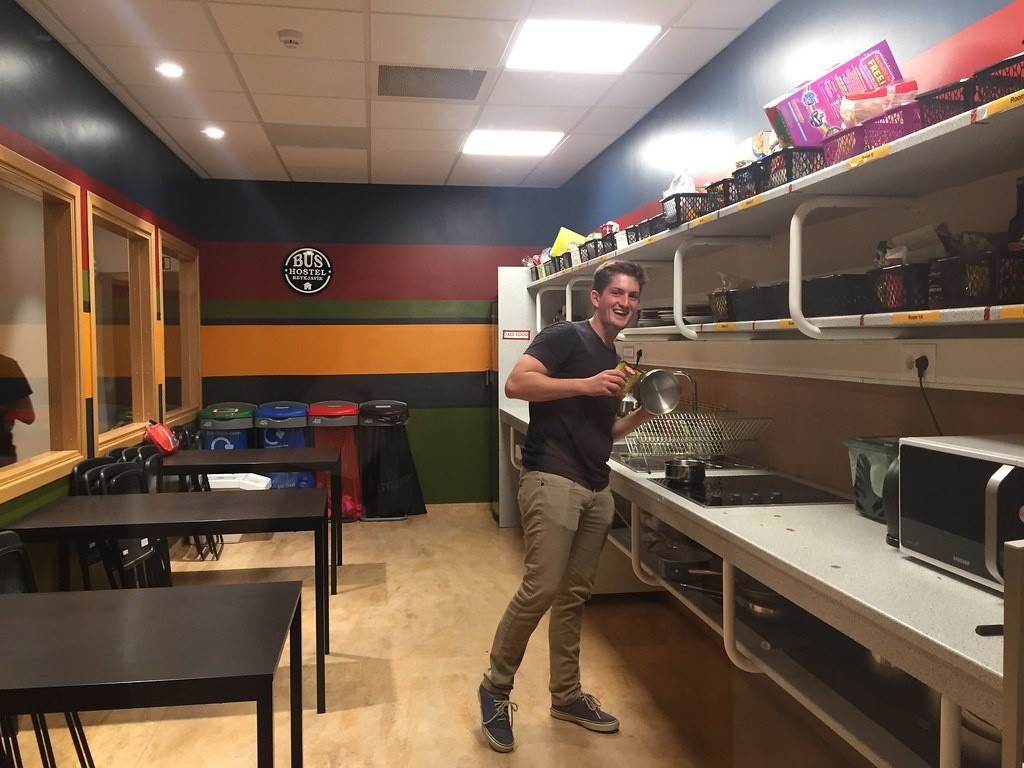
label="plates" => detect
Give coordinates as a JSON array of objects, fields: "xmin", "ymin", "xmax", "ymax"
[
  {"xmin": 682, "ymin": 304, "xmax": 712, "ymax": 323},
  {"xmin": 637, "ymin": 308, "xmax": 674, "ymax": 327}
]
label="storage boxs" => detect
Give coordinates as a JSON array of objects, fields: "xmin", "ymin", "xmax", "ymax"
[
  {"xmin": 843, "ymin": 434, "xmax": 913, "ymax": 524},
  {"xmin": 530, "ymin": 39, "xmax": 1024, "ymax": 322}
]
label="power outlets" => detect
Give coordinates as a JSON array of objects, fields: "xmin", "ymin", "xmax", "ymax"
[
  {"xmin": 633, "ymin": 344, "xmax": 647, "ymax": 364},
  {"xmin": 900, "ymin": 344, "xmax": 937, "ymax": 383}
]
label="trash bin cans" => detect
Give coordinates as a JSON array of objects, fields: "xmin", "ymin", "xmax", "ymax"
[
  {"xmin": 199, "ymin": 402, "xmax": 257, "ymax": 449},
  {"xmin": 308, "ymin": 400, "xmax": 359, "ymax": 523},
  {"xmin": 254, "ymin": 400, "xmax": 313, "ymax": 490},
  {"xmin": 358, "ymin": 399, "xmax": 428, "ymax": 521}
]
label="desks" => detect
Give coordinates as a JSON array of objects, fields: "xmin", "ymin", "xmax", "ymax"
[
  {"xmin": 165, "ymin": 446, "xmax": 344, "ymax": 595},
  {"xmin": 1, "ymin": 486, "xmax": 329, "ymax": 715},
  {"xmin": 0, "ymin": 582, "xmax": 303, "ymax": 768}
]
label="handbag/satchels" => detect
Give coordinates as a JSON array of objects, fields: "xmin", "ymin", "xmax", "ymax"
[{"xmin": 143, "ymin": 419, "xmax": 180, "ymax": 455}]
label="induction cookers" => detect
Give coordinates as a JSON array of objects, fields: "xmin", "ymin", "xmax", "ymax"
[{"xmin": 649, "ymin": 475, "xmax": 852, "ymax": 513}]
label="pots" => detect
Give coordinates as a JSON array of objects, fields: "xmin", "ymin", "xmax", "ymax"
[
  {"xmin": 688, "ymin": 569, "xmax": 776, "ymax": 607},
  {"xmin": 665, "ymin": 460, "xmax": 706, "ymax": 483},
  {"xmin": 656, "ymin": 547, "xmax": 713, "ymax": 580},
  {"xmin": 682, "ymin": 583, "xmax": 789, "ymax": 622}
]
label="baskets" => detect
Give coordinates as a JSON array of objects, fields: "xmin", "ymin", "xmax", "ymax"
[{"xmin": 530, "ymin": 52, "xmax": 1024, "ymax": 322}]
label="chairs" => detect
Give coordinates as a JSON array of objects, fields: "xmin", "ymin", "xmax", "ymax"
[
  {"xmin": 0, "ymin": 529, "xmax": 95, "ymax": 768},
  {"xmin": 73, "ymin": 418, "xmax": 224, "ymax": 591}
]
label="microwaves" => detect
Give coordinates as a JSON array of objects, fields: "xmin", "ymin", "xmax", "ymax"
[{"xmin": 899, "ymin": 434, "xmax": 1024, "ymax": 594}]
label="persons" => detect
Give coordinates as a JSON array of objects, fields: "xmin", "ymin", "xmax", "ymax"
[
  {"xmin": 0, "ymin": 353, "xmax": 36, "ymax": 468},
  {"xmin": 477, "ymin": 259, "xmax": 659, "ymax": 755}
]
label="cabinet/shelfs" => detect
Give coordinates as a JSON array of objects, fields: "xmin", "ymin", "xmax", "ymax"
[{"xmin": 496, "ymin": 89, "xmax": 1024, "ymax": 768}]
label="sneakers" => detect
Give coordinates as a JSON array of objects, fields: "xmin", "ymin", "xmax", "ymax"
[
  {"xmin": 477, "ymin": 683, "xmax": 518, "ymax": 752},
  {"xmin": 550, "ymin": 692, "xmax": 619, "ymax": 732}
]
